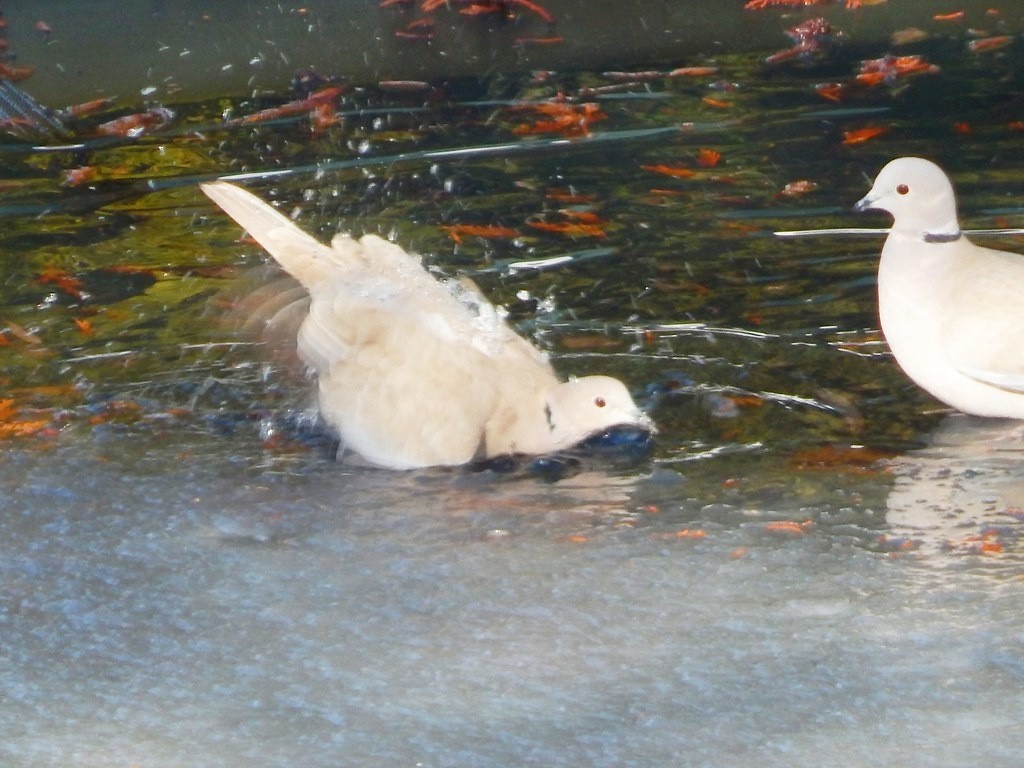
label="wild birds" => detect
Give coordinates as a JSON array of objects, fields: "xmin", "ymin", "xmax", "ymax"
[
  {"xmin": 845, "ymin": 151, "xmax": 1024, "ymax": 424},
  {"xmin": 199, "ymin": 178, "xmax": 656, "ymax": 469}
]
